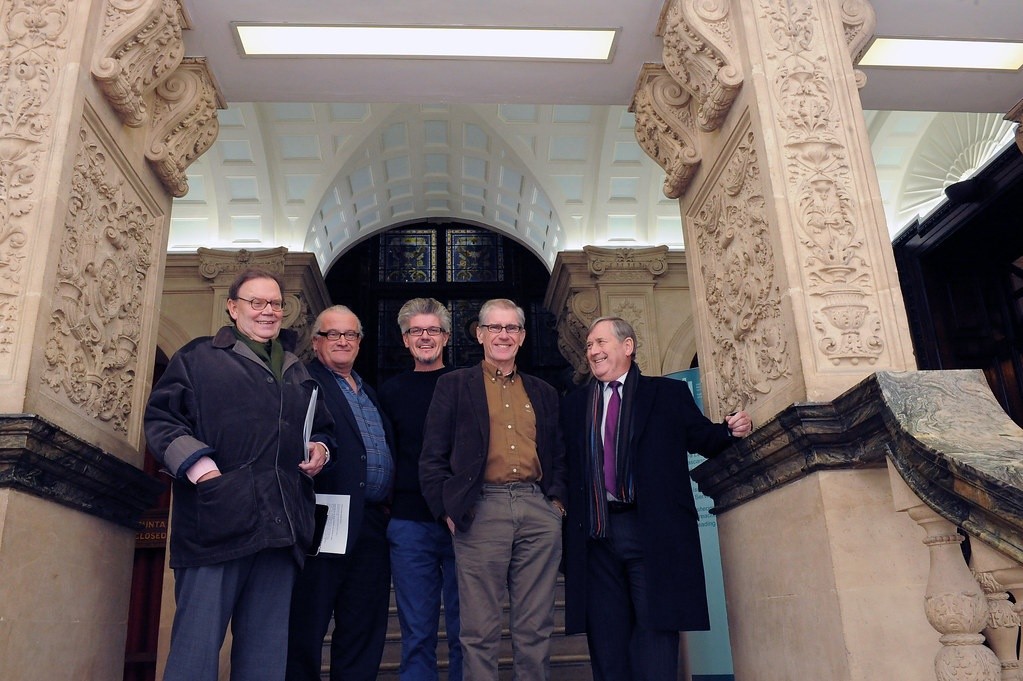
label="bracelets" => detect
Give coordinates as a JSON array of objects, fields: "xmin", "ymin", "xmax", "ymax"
[
  {"xmin": 553, "ymin": 501, "xmax": 567, "ymax": 518},
  {"xmin": 321, "ymin": 444, "xmax": 328, "ymax": 466}
]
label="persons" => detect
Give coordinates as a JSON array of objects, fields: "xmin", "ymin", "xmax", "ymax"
[
  {"xmin": 374, "ymin": 297, "xmax": 461, "ymax": 681},
  {"xmin": 143, "ymin": 270, "xmax": 339, "ymax": 681},
  {"xmin": 285, "ymin": 304, "xmax": 400, "ymax": 681},
  {"xmin": 419, "ymin": 303, "xmax": 570, "ymax": 681},
  {"xmin": 559, "ymin": 316, "xmax": 754, "ymax": 681}
]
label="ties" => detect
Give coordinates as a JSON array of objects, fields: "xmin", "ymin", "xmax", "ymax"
[{"xmin": 604, "ymin": 380, "xmax": 622, "ymax": 498}]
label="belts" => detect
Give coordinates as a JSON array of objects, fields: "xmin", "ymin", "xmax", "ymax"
[{"xmin": 606, "ymin": 501, "xmax": 635, "ymax": 513}]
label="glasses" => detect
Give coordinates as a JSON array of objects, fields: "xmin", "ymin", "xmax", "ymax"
[
  {"xmin": 237, "ymin": 296, "xmax": 287, "ymax": 312},
  {"xmin": 316, "ymin": 329, "xmax": 359, "ymax": 341},
  {"xmin": 403, "ymin": 326, "xmax": 446, "ymax": 335},
  {"xmin": 480, "ymin": 324, "xmax": 523, "ymax": 333}
]
